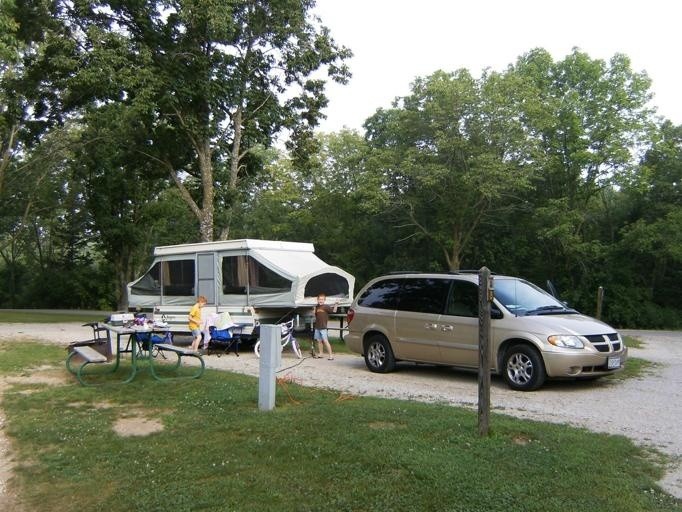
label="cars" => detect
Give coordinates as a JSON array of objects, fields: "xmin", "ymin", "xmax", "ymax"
[{"xmin": 123, "ymin": 319, "xmax": 128, "ymax": 329}]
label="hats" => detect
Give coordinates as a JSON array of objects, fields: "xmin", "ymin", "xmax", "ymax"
[{"xmin": 344, "ymin": 270, "xmax": 628, "ymax": 391}]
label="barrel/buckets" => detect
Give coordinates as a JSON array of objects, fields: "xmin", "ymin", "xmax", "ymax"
[
  {"xmin": 328, "ymin": 356, "xmax": 334, "ymax": 360},
  {"xmin": 314, "ymin": 353, "xmax": 324, "ymax": 359}
]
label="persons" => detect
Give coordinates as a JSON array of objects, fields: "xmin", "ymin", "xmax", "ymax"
[
  {"xmin": 312, "ymin": 293, "xmax": 338, "ymax": 360},
  {"xmin": 187, "ymin": 295, "xmax": 207, "ymax": 356}
]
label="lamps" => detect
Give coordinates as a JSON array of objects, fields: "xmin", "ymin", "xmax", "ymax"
[
  {"xmin": 135, "ymin": 333, "xmax": 169, "ymax": 359},
  {"xmin": 208, "ymin": 312, "xmax": 246, "ymax": 359}
]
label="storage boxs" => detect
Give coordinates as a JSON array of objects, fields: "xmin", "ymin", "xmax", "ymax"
[{"xmin": 69, "ymin": 342, "xmax": 205, "ymax": 385}]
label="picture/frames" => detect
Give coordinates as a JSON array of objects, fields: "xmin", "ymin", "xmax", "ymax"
[{"xmin": 101, "ymin": 322, "xmax": 171, "ymax": 384}]
